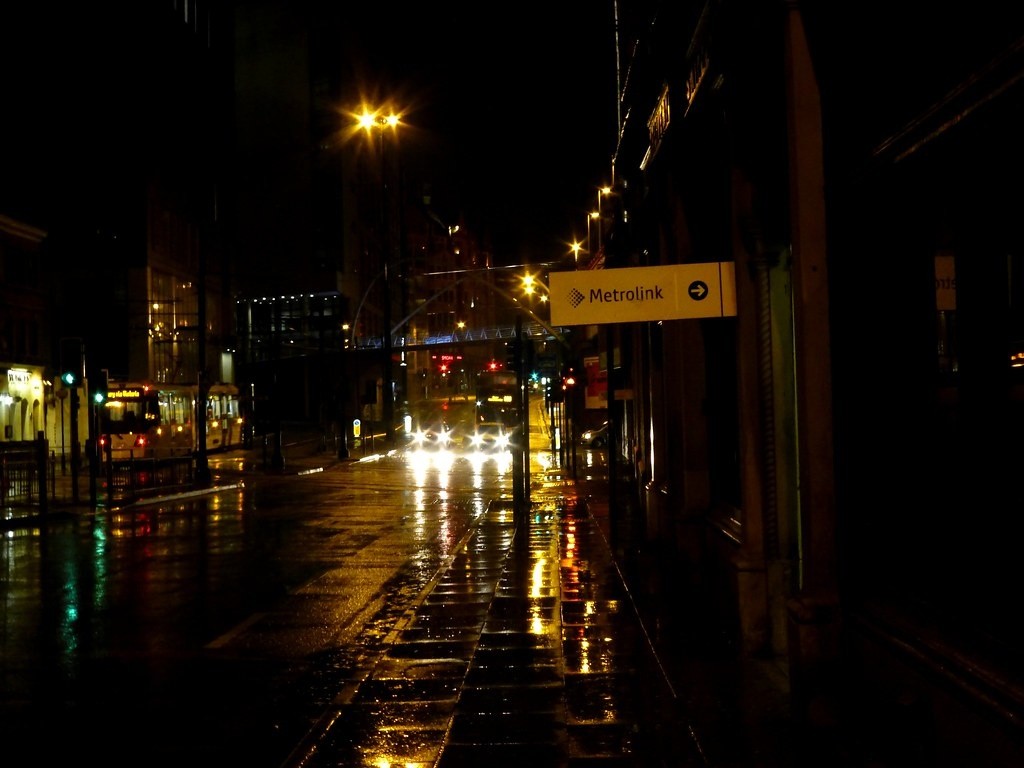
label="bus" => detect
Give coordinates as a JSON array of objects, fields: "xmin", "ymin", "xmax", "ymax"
[
  {"xmin": 104, "ymin": 381, "xmax": 242, "ymax": 465},
  {"xmin": 474, "ymin": 371, "xmax": 523, "ymax": 432}
]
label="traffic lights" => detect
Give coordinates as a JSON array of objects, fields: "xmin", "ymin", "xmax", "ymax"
[
  {"xmin": 60, "ymin": 372, "xmax": 78, "ymax": 387},
  {"xmin": 91, "ymin": 392, "xmax": 106, "ymax": 406}
]
